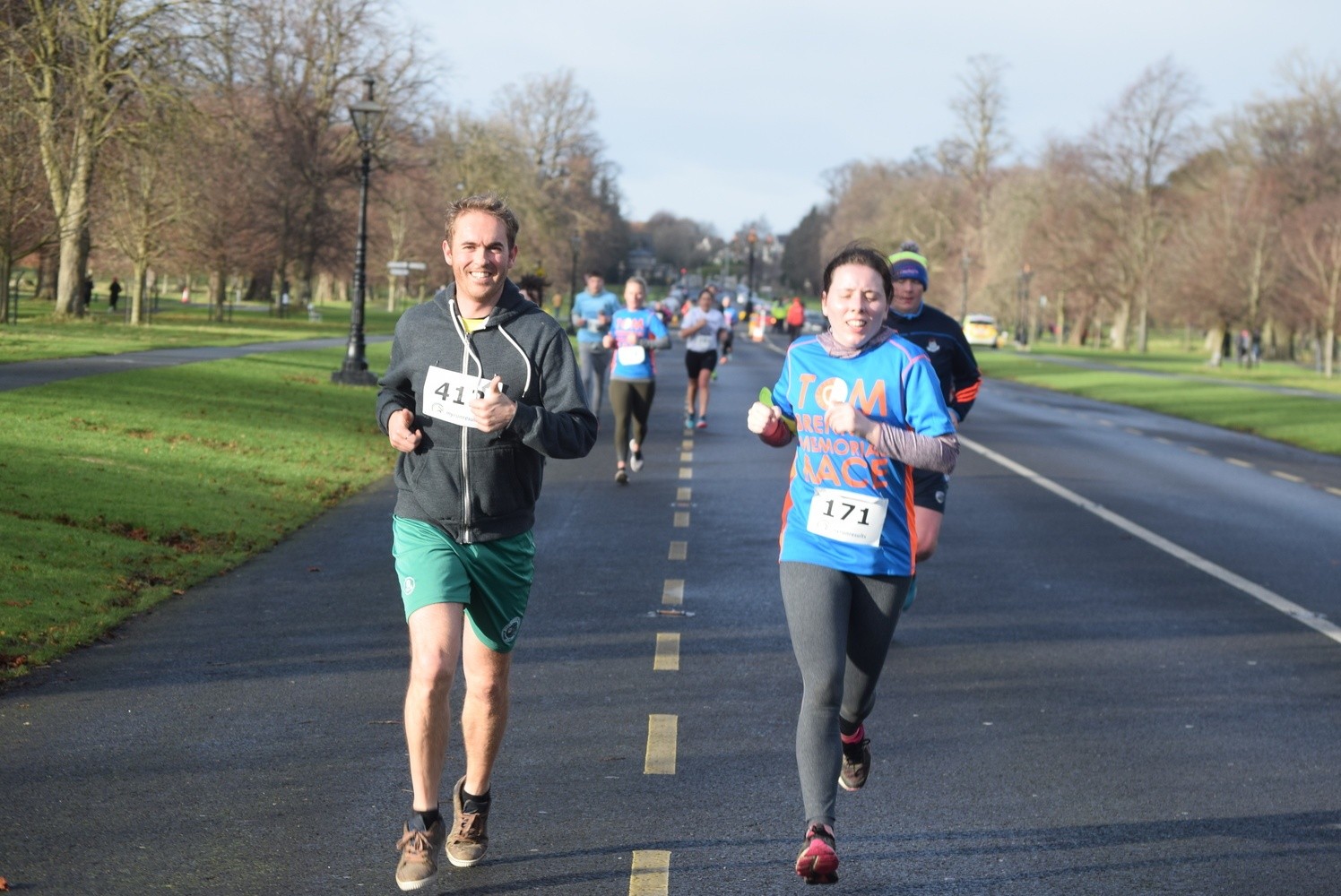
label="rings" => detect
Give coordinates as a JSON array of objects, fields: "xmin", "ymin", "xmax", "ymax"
[{"xmin": 405, "ymin": 433, "xmax": 412, "ymax": 439}]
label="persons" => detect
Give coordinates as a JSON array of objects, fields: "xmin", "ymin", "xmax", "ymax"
[
  {"xmin": 81, "ymin": 274, "xmax": 95, "ymax": 311},
  {"xmin": 375, "ymin": 190, "xmax": 598, "ymax": 891},
  {"xmin": 1201, "ymin": 318, "xmax": 1264, "ymax": 370},
  {"xmin": 883, "ymin": 240, "xmax": 983, "ymax": 613},
  {"xmin": 745, "ymin": 247, "xmax": 961, "ymax": 886},
  {"xmin": 513, "ymin": 270, "xmax": 806, "ymax": 486},
  {"xmin": 107, "ymin": 274, "xmax": 122, "ymax": 313}
]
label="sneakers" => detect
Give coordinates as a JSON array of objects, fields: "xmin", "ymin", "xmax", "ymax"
[
  {"xmin": 395, "ymin": 814, "xmax": 445, "ymax": 890},
  {"xmin": 445, "ymin": 774, "xmax": 491, "ymax": 868},
  {"xmin": 902, "ymin": 564, "xmax": 918, "ymax": 610},
  {"xmin": 838, "ymin": 725, "xmax": 871, "ymax": 792},
  {"xmin": 795, "ymin": 824, "xmax": 839, "ymax": 884},
  {"xmin": 614, "ymin": 468, "xmax": 628, "ymax": 486},
  {"xmin": 629, "ymin": 438, "xmax": 645, "ymax": 473}
]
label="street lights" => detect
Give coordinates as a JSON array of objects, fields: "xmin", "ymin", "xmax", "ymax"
[
  {"xmin": 329, "ymin": 78, "xmax": 388, "ymax": 387},
  {"xmin": 743, "ymin": 227, "xmax": 757, "ymax": 322},
  {"xmin": 565, "ymin": 229, "xmax": 583, "ymax": 337}
]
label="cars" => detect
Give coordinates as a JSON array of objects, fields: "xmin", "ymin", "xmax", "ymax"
[{"xmin": 961, "ymin": 314, "xmax": 999, "ymax": 348}]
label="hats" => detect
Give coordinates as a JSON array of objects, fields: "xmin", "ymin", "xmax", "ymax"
[{"xmin": 884, "ymin": 240, "xmax": 929, "ymax": 291}]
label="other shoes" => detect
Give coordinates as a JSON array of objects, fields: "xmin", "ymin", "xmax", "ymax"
[
  {"xmin": 696, "ymin": 415, "xmax": 707, "ymax": 429},
  {"xmin": 684, "ymin": 413, "xmax": 694, "ymax": 429}
]
